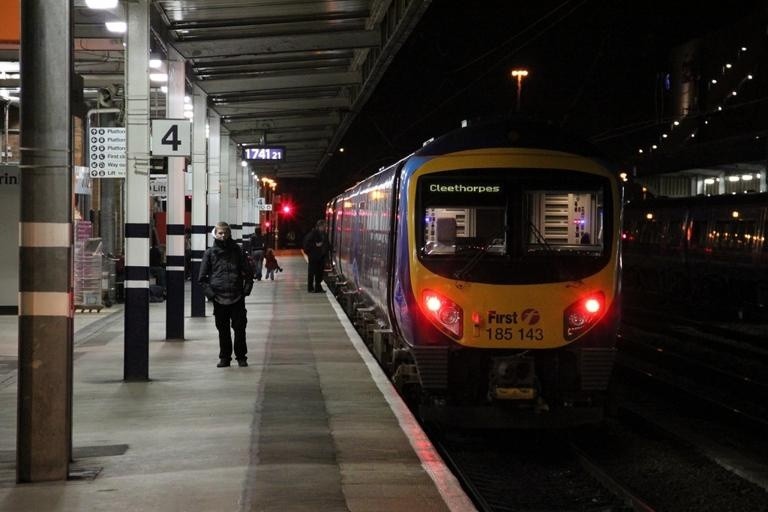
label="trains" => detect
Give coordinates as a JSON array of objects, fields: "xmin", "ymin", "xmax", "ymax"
[
  {"xmin": 619, "ymin": 191, "xmax": 767, "ymax": 328},
  {"xmin": 321, "ymin": 113, "xmax": 631, "ymax": 434}
]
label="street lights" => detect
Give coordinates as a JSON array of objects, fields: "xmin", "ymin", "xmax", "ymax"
[{"xmin": 511, "ymin": 70, "xmax": 528, "ymax": 112}]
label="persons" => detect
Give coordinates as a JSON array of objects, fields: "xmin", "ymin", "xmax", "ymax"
[
  {"xmin": 302, "ymin": 219, "xmax": 334, "ymax": 293},
  {"xmin": 263, "ymin": 248, "xmax": 278, "ymax": 280},
  {"xmin": 198, "ymin": 221, "xmax": 253, "ymax": 368},
  {"xmin": 262, "ymin": 226, "xmax": 276, "ymax": 254},
  {"xmin": 249, "ymin": 227, "xmax": 265, "ymax": 280}
]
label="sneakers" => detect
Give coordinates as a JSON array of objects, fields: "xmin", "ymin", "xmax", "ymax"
[
  {"xmin": 217, "ymin": 359, "xmax": 231, "ymax": 367},
  {"xmin": 238, "ymin": 359, "xmax": 248, "ymax": 366},
  {"xmin": 315, "ymin": 289, "xmax": 326, "ymax": 293},
  {"xmin": 309, "ymin": 288, "xmax": 314, "ymax": 292}
]
